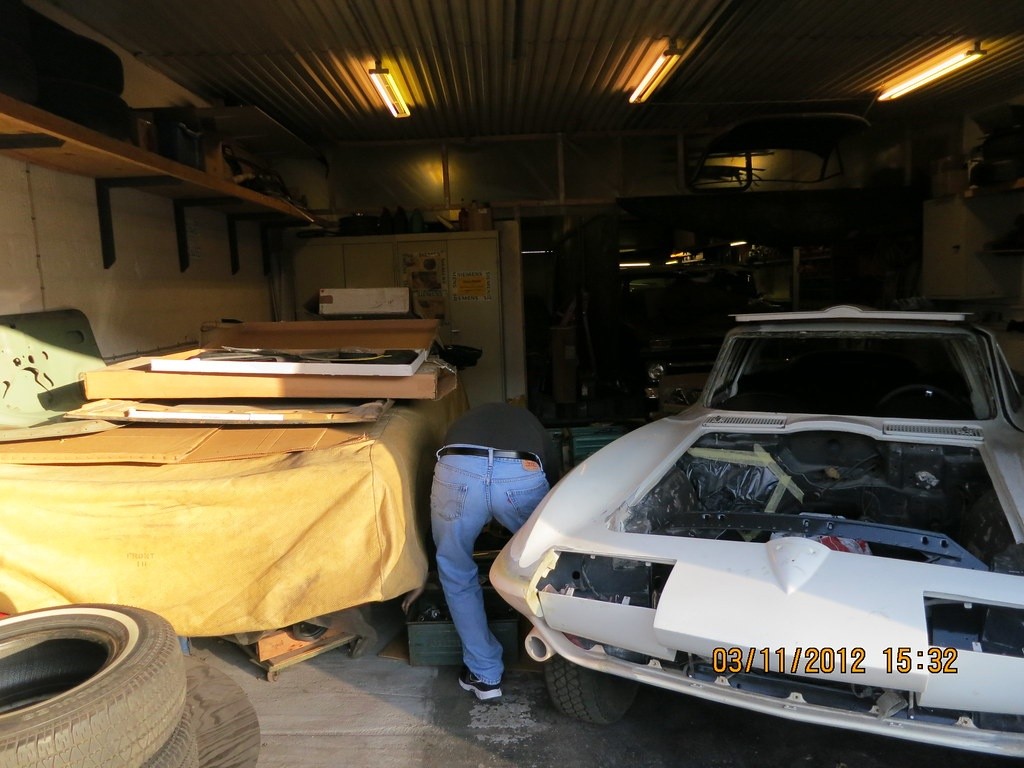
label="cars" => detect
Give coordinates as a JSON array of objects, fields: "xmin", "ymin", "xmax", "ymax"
[
  {"xmin": 488, "ymin": 303, "xmax": 1024, "ymax": 760},
  {"xmin": 590, "ymin": 265, "xmax": 764, "ymax": 419}
]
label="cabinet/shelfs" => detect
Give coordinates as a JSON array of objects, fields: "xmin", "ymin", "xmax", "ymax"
[
  {"xmin": 916, "ymin": 175, "xmax": 1024, "ymax": 312},
  {"xmin": 1, "ymin": 0, "xmax": 334, "ymax": 230},
  {"xmin": 291, "ymin": 229, "xmax": 508, "ymax": 411}
]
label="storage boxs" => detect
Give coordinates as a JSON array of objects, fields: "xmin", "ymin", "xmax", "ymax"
[{"xmin": 318, "ymin": 287, "xmax": 410, "ymax": 316}]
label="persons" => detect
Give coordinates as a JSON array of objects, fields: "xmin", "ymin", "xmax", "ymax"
[{"xmin": 427, "ymin": 400, "xmax": 561, "ymax": 704}]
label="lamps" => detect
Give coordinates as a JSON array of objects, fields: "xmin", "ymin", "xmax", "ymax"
[
  {"xmin": 877, "ymin": 37, "xmax": 988, "ymax": 100},
  {"xmin": 629, "ymin": 37, "xmax": 684, "ymax": 104},
  {"xmin": 367, "ymin": 61, "xmax": 411, "ymax": 119}
]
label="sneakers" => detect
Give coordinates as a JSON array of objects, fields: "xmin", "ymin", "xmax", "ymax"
[{"xmin": 458, "ymin": 670, "xmax": 502, "ymax": 701}]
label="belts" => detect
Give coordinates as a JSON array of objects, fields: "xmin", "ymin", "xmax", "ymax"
[{"xmin": 437, "ymin": 447, "xmax": 543, "ymax": 467}]
